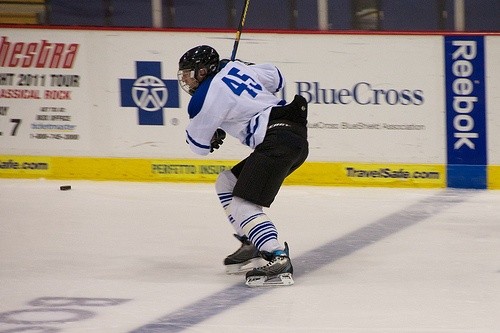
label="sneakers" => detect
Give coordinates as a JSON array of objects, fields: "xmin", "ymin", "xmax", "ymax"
[
  {"xmin": 223, "ymin": 234, "xmax": 271, "ymax": 274},
  {"xmin": 246, "ymin": 242, "xmax": 294, "ymax": 287}
]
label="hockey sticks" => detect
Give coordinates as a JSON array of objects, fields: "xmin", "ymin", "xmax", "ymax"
[{"xmin": 230, "ymin": 0, "xmax": 250, "ymax": 63}]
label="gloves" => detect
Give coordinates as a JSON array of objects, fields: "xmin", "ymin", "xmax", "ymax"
[{"xmin": 209, "ymin": 128, "xmax": 226, "ymax": 153}]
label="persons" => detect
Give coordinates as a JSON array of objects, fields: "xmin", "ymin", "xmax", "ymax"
[{"xmin": 177, "ymin": 46, "xmax": 309, "ymax": 277}]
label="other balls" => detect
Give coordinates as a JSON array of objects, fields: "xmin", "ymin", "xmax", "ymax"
[{"xmin": 60, "ymin": 185, "xmax": 71, "ymax": 191}]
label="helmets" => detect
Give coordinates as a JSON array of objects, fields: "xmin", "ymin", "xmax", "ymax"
[{"xmin": 179, "ymin": 44, "xmax": 219, "ymax": 80}]
